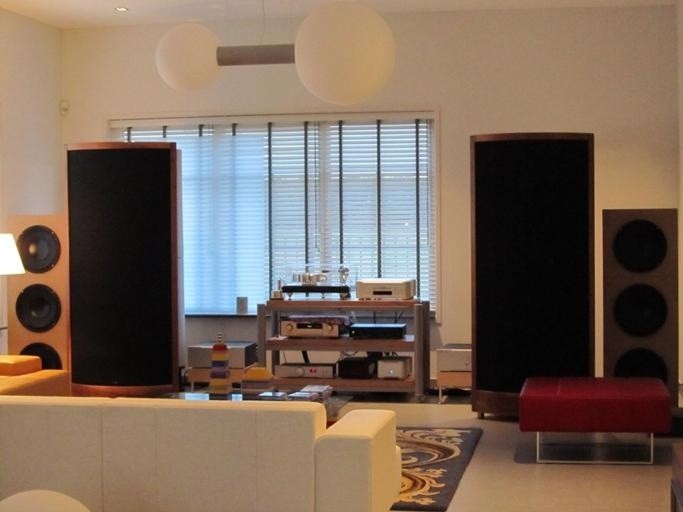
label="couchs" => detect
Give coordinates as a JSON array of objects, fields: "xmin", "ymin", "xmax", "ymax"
[{"xmin": 0, "ymin": 353, "xmax": 402, "ymax": 511}]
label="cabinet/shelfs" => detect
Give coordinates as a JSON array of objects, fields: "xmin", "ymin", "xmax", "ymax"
[
  {"xmin": 188, "ymin": 369, "xmax": 245, "ymax": 393},
  {"xmin": 436, "ymin": 344, "xmax": 472, "ymax": 404},
  {"xmin": 255, "ymin": 296, "xmax": 432, "ymax": 402}
]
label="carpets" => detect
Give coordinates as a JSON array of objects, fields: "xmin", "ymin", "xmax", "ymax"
[{"xmin": 391, "ymin": 426, "xmax": 483, "ymax": 511}]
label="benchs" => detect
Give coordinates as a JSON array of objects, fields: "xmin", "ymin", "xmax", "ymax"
[{"xmin": 518, "ymin": 376, "xmax": 673, "ymax": 465}]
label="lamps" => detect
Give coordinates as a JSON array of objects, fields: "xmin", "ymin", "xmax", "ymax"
[
  {"xmin": 154, "ymin": 0, "xmax": 399, "ymax": 108},
  {"xmin": 0, "ymin": 233, "xmax": 26, "ymax": 275}
]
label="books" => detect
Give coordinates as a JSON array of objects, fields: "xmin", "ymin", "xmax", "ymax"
[{"xmin": 257, "ymin": 383, "xmax": 333, "ymax": 407}]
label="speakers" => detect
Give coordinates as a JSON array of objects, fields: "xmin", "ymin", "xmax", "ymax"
[
  {"xmin": 471, "ymin": 133, "xmax": 594, "ymax": 419},
  {"xmin": 67, "ymin": 140, "xmax": 186, "ymax": 396},
  {"xmin": 602, "ymin": 209, "xmax": 678, "ymax": 407},
  {"xmin": 7, "ymin": 213, "xmax": 69, "ymax": 370}
]
